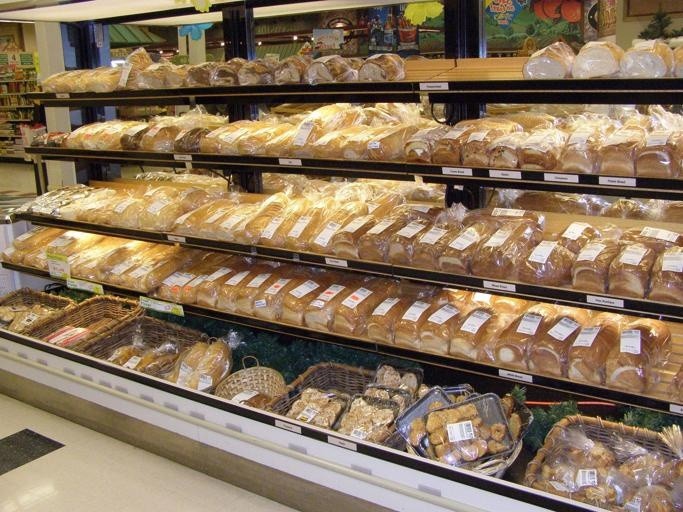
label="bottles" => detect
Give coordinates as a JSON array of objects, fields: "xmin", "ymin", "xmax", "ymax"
[{"xmin": 383, "ymin": 14, "xmax": 393, "ymax": 51}]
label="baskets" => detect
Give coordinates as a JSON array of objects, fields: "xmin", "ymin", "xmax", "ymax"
[
  {"xmin": 523, "ymin": 411, "xmax": 683, "ymax": 511},
  {"xmin": 405, "ymin": 432, "xmax": 524, "ymax": 481},
  {"xmin": 20, "ymin": 294, "xmax": 142, "ymax": 350},
  {"xmin": 213, "ymin": 355, "xmax": 289, "ymax": 416},
  {"xmin": 69, "ymin": 316, "xmax": 209, "ymax": 377},
  {"xmin": 263, "ymin": 359, "xmax": 409, "ymax": 452},
  {"xmin": 0, "ymin": 286, "xmax": 76, "ymax": 333}
]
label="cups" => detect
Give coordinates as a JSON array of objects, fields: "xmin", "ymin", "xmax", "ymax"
[{"xmin": 397, "ymin": 25, "xmax": 418, "ymax": 45}]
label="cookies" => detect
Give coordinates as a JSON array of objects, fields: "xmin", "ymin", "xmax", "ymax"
[{"xmin": 286, "ymin": 364, "xmax": 521, "ymax": 464}]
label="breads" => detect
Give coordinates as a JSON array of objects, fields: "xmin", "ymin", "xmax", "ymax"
[
  {"xmin": 0, "ymin": 103, "xmax": 683, "ymax": 394},
  {"xmin": 673, "ymin": 44, "xmax": 683, "ymax": 77},
  {"xmin": 522, "ymin": 42, "xmax": 575, "ymax": 80},
  {"xmin": 542, "ymin": 447, "xmax": 683, "ymax": 512},
  {"xmin": 620, "ymin": 40, "xmax": 672, "ymax": 78},
  {"xmin": 41, "ymin": 48, "xmax": 404, "ymax": 93},
  {"xmin": 571, "ymin": 40, "xmax": 624, "ymax": 79}
]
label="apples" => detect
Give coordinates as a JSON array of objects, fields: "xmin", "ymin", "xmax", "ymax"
[{"xmin": 534, "ymin": 0, "xmax": 581, "ymax": 22}]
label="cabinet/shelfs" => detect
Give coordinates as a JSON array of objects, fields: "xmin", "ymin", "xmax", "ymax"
[{"xmin": 0, "ymin": 77, "xmax": 683, "ymax": 512}]
